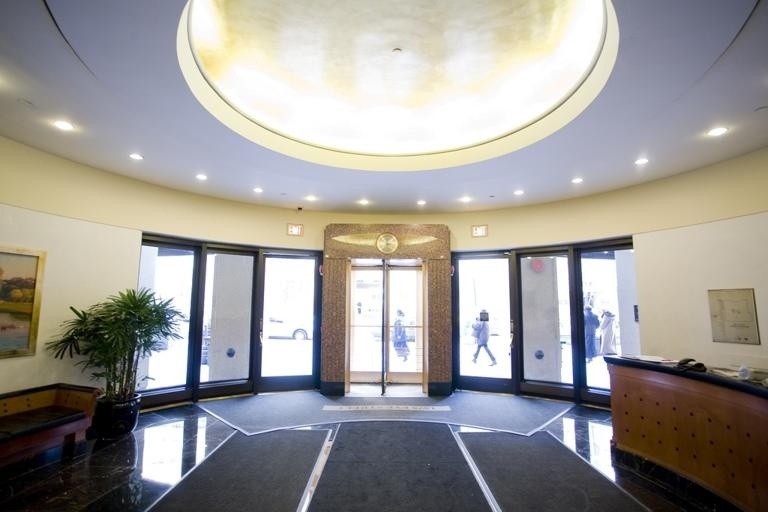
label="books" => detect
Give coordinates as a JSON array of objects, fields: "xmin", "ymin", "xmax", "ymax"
[{"xmin": 619, "ymin": 351, "xmax": 679, "ymax": 364}]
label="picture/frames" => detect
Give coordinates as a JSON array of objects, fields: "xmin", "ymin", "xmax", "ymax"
[{"xmin": 0, "ymin": 244, "xmax": 48, "ymax": 360}]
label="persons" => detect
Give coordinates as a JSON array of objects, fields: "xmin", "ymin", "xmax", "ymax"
[
  {"xmin": 471, "ymin": 308, "xmax": 497, "ymax": 367},
  {"xmin": 599, "ymin": 309, "xmax": 617, "ymax": 355},
  {"xmin": 584, "ymin": 305, "xmax": 601, "ymax": 363},
  {"xmin": 390, "ymin": 309, "xmax": 410, "ymax": 363},
  {"xmin": 472, "ymin": 317, "xmax": 481, "ymax": 345}
]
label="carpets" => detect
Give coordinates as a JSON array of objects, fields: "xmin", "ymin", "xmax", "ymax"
[
  {"xmin": 452, "ymin": 429, "xmax": 654, "ymax": 512},
  {"xmin": 192, "ymin": 388, "xmax": 576, "ymax": 437},
  {"xmin": 139, "ymin": 427, "xmax": 333, "ymax": 512},
  {"xmin": 305, "ymin": 421, "xmax": 496, "ymax": 512}
]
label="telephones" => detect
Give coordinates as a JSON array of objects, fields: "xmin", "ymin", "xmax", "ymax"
[{"xmin": 677, "ymin": 358, "xmax": 696, "ymax": 368}]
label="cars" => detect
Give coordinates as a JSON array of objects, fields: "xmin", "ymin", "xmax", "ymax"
[
  {"xmin": 560, "ymin": 316, "xmax": 619, "ymax": 346},
  {"xmin": 263, "ymin": 303, "xmax": 312, "ymax": 340},
  {"xmin": 373, "ymin": 317, "xmax": 416, "ymax": 341}
]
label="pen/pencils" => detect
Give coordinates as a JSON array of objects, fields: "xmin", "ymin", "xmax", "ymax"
[{"xmin": 661, "ymin": 360, "xmax": 673, "ymax": 361}]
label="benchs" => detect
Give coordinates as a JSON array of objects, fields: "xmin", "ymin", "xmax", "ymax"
[{"xmin": 0, "ymin": 382, "xmax": 102, "ymax": 468}]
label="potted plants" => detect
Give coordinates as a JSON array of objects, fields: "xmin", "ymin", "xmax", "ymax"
[
  {"xmin": 90, "ymin": 433, "xmax": 145, "ymax": 511},
  {"xmin": 44, "ymin": 285, "xmax": 187, "ymax": 435}
]
